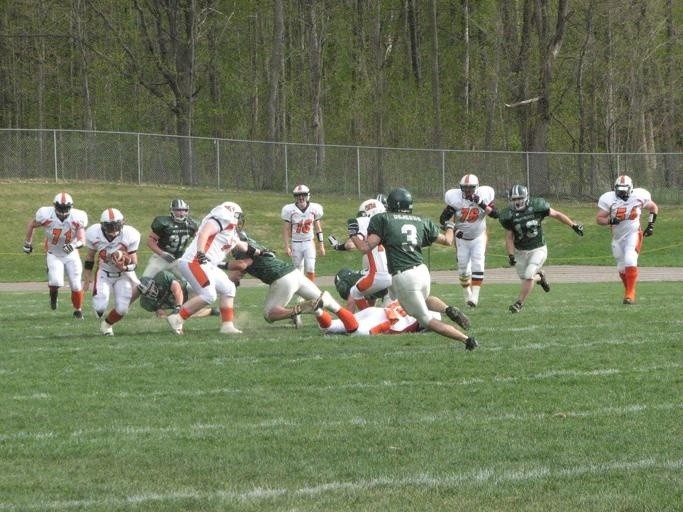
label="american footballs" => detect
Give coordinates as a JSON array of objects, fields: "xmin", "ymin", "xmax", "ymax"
[{"xmin": 110, "ymin": 250, "xmax": 132, "ymax": 266}]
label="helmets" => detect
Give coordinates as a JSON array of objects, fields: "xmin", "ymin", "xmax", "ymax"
[
  {"xmin": 138, "ymin": 278, "xmax": 158, "ymax": 298},
  {"xmin": 335, "ymin": 269, "xmax": 354, "ymax": 299}
]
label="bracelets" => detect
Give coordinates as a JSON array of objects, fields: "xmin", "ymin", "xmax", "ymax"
[{"xmin": 316, "ymin": 232, "xmax": 324, "ymax": 243}]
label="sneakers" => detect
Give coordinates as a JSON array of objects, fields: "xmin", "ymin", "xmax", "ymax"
[
  {"xmin": 72, "ymin": 310, "xmax": 84, "ymax": 320},
  {"xmin": 510, "ymin": 302, "xmax": 523, "ymax": 313},
  {"xmin": 167, "ymin": 314, "xmax": 184, "ymax": 335},
  {"xmin": 447, "ymin": 307, "xmax": 471, "ymax": 329},
  {"xmin": 51, "ymin": 298, "xmax": 58, "ymax": 309},
  {"xmin": 298, "ymin": 300, "xmax": 316, "ymax": 312},
  {"xmin": 220, "ymin": 323, "xmax": 242, "ymax": 334},
  {"xmin": 95, "ymin": 310, "xmax": 104, "ymax": 318},
  {"xmin": 100, "ymin": 322, "xmax": 114, "ymax": 336},
  {"xmin": 623, "ymin": 298, "xmax": 632, "ymax": 303},
  {"xmin": 537, "ymin": 273, "xmax": 550, "ymax": 292},
  {"xmin": 469, "ymin": 297, "xmax": 476, "ymax": 306},
  {"xmin": 463, "ymin": 291, "xmax": 471, "ymax": 303},
  {"xmin": 465, "ymin": 337, "xmax": 478, "ymax": 352},
  {"xmin": 314, "ymin": 290, "xmax": 330, "ymax": 311},
  {"xmin": 211, "ymin": 307, "xmax": 220, "ymax": 315},
  {"xmin": 290, "ymin": 313, "xmax": 301, "ymax": 329}
]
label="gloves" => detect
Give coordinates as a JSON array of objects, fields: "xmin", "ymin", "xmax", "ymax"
[
  {"xmin": 260, "ymin": 249, "xmax": 275, "ymax": 257},
  {"xmin": 197, "ymin": 251, "xmax": 209, "ymax": 265},
  {"xmin": 163, "ymin": 252, "xmax": 174, "ymax": 262},
  {"xmin": 509, "ymin": 255, "xmax": 516, "ymax": 265},
  {"xmin": 643, "ymin": 224, "xmax": 653, "ymax": 237},
  {"xmin": 328, "ymin": 234, "xmax": 339, "ymax": 249},
  {"xmin": 171, "ymin": 305, "xmax": 181, "ymax": 313},
  {"xmin": 63, "ymin": 244, "xmax": 72, "ymax": 253},
  {"xmin": 22, "ymin": 241, "xmax": 33, "ymax": 253}
]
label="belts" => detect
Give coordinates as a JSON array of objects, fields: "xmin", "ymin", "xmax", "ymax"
[{"xmin": 392, "ymin": 264, "xmax": 421, "ymax": 276}]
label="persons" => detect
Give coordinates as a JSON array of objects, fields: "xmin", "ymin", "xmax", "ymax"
[
  {"xmin": 82, "ymin": 209, "xmax": 142, "ymax": 336},
  {"xmin": 168, "ymin": 202, "xmax": 277, "ymax": 335},
  {"xmin": 439, "ymin": 174, "xmax": 499, "ymax": 308},
  {"xmin": 347, "ymin": 187, "xmax": 479, "ymax": 351},
  {"xmin": 596, "ymin": 174, "xmax": 658, "ymax": 304},
  {"xmin": 23, "ymin": 191, "xmax": 88, "ymax": 320},
  {"xmin": 126, "ymin": 199, "xmax": 199, "ymax": 308},
  {"xmin": 218, "ymin": 229, "xmax": 358, "ymax": 334},
  {"xmin": 499, "ymin": 183, "xmax": 586, "ymax": 313},
  {"xmin": 134, "ymin": 267, "xmax": 240, "ymax": 320},
  {"xmin": 321, "ymin": 191, "xmax": 443, "ymax": 338},
  {"xmin": 279, "ymin": 183, "xmax": 328, "ymax": 305}
]
